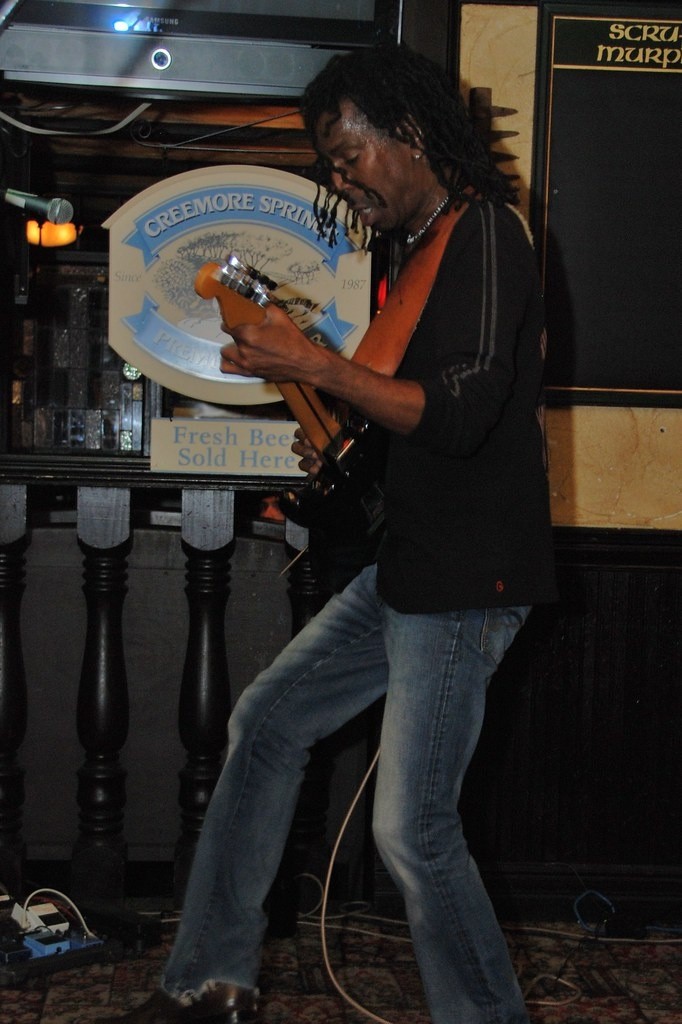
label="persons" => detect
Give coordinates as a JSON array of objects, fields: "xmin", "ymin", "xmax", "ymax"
[{"xmin": 91, "ymin": 44, "xmax": 556, "ymax": 1024}]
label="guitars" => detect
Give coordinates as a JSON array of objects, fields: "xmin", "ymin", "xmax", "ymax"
[{"xmin": 193, "ymin": 252, "xmax": 386, "ymax": 636}]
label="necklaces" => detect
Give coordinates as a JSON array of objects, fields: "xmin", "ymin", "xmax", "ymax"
[{"xmin": 407, "ymin": 195, "xmax": 449, "ymax": 245}]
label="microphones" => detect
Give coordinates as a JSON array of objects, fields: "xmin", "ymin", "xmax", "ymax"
[{"xmin": 0, "ymin": 186, "xmax": 74, "ymax": 224}]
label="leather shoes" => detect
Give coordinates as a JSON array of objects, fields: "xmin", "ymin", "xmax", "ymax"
[{"xmin": 93, "ymin": 981, "xmax": 261, "ymax": 1024}]
